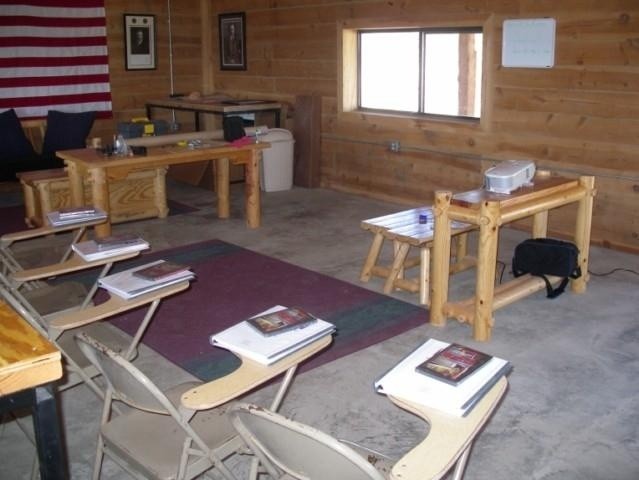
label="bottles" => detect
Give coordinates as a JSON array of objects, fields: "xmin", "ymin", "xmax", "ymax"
[{"xmin": 116, "ymin": 135, "xmax": 128, "ymax": 155}]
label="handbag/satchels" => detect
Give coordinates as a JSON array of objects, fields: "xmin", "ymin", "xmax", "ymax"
[
  {"xmin": 513, "ymin": 238, "xmax": 582, "ymax": 279},
  {"xmin": 222, "ymin": 116, "xmax": 246, "ymax": 142}
]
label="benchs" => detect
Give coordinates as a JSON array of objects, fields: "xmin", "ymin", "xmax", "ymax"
[
  {"xmin": 15, "ymin": 164, "xmax": 171, "ymax": 238},
  {"xmin": 0, "ymin": 119, "xmax": 48, "ymax": 209}
]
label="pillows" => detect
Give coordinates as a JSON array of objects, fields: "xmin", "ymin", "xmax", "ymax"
[
  {"xmin": 0, "ymin": 108, "xmax": 53, "ymax": 182},
  {"xmin": 36, "ymin": 111, "xmax": 95, "ymax": 169}
]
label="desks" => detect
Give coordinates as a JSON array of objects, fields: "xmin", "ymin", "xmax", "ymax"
[
  {"xmin": 144, "ymin": 96, "xmax": 282, "ymax": 133},
  {"xmin": 56, "ymin": 136, "xmax": 272, "ymax": 242},
  {"xmin": 430, "ymin": 168, "xmax": 596, "ymax": 342}
]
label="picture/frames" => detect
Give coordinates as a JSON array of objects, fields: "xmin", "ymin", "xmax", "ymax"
[
  {"xmin": 123, "ymin": 14, "xmax": 156, "ymax": 70},
  {"xmin": 218, "ymin": 12, "xmax": 247, "ymax": 71}
]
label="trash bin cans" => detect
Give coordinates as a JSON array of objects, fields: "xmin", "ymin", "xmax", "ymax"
[
  {"xmin": 259, "ymin": 127, "xmax": 296, "ymax": 192},
  {"xmin": 118, "ymin": 118, "xmax": 168, "ymax": 154}
]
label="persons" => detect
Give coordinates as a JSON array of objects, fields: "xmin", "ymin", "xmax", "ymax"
[
  {"xmin": 223, "ymin": 23, "xmax": 241, "ymax": 63},
  {"xmin": 132, "ymin": 29, "xmax": 148, "ymax": 53}
]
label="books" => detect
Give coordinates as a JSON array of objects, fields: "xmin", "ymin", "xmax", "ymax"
[
  {"xmin": 208, "ymin": 304, "xmax": 337, "ymax": 367},
  {"xmin": 44, "ymin": 205, "xmax": 196, "ymax": 301},
  {"xmin": 374, "ymin": 338, "xmax": 513, "ymax": 418}
]
